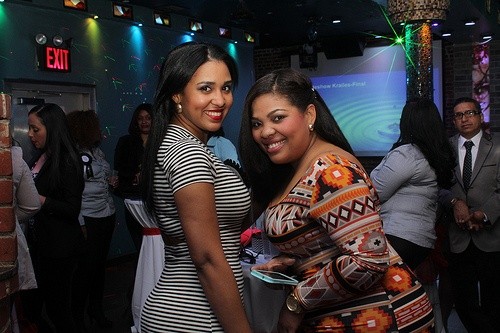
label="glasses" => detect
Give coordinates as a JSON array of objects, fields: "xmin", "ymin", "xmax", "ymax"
[{"xmin": 453, "ymin": 110, "xmax": 483, "ymax": 121}]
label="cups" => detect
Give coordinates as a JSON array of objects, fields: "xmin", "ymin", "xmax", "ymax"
[{"xmin": 110, "ymin": 170, "xmax": 119, "ymax": 188}]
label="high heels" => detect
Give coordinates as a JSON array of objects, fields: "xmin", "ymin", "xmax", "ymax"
[{"xmin": 86, "ymin": 304, "xmax": 114, "ymax": 329}]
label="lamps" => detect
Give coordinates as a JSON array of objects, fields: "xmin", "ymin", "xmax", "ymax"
[{"xmin": 30, "ymin": 32, "xmax": 72, "ymax": 74}]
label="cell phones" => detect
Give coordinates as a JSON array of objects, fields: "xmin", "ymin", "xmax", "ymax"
[{"xmin": 252, "ymin": 270, "xmax": 299, "ymax": 284}]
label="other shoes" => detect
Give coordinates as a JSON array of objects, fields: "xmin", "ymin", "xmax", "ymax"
[
  {"xmin": 47, "ymin": 311, "xmax": 73, "ymax": 333},
  {"xmin": 28, "ymin": 317, "xmax": 54, "ymax": 333}
]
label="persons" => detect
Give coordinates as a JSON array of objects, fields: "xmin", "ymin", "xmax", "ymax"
[
  {"xmin": 416, "ymin": 203, "xmax": 459, "ymax": 333},
  {"xmin": 11, "ymin": 135, "xmax": 42, "ymax": 333},
  {"xmin": 66, "ymin": 110, "xmax": 119, "ymax": 333},
  {"xmin": 22, "ymin": 103, "xmax": 90, "ymax": 333},
  {"xmin": 114, "ymin": 103, "xmax": 153, "ymax": 323},
  {"xmin": 140, "ymin": 42, "xmax": 255, "ymax": 333},
  {"xmin": 440, "ymin": 97, "xmax": 500, "ymax": 333},
  {"xmin": 370, "ymin": 97, "xmax": 458, "ymax": 292},
  {"xmin": 238, "ymin": 70, "xmax": 435, "ymax": 333}
]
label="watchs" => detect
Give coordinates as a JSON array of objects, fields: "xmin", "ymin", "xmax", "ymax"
[
  {"xmin": 451, "ymin": 199, "xmax": 457, "ymax": 205},
  {"xmin": 286, "ymin": 293, "xmax": 304, "ymax": 313}
]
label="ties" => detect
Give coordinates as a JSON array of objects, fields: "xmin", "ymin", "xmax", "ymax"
[{"xmin": 462, "ymin": 142, "xmax": 471, "ymax": 209}]
